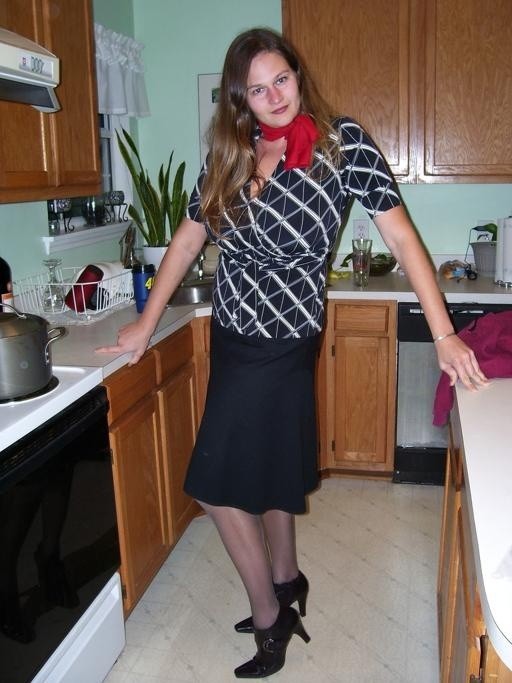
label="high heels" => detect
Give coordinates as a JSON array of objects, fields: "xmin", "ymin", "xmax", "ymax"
[
  {"xmin": 235, "ymin": 570, "xmax": 308, "ymax": 633},
  {"xmin": 235, "ymin": 606, "xmax": 311, "ymax": 678}
]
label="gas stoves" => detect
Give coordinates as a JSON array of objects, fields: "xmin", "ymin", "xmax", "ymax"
[{"xmin": 0, "ymin": 366, "xmax": 104, "ymax": 450}]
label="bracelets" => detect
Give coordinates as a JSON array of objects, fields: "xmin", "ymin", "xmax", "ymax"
[{"xmin": 433, "ymin": 332, "xmax": 457, "ymax": 345}]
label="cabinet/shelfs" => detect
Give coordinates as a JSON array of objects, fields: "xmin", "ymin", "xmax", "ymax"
[
  {"xmin": 438, "ymin": 390, "xmax": 512, "ymax": 683},
  {"xmin": 281, "ymin": 1, "xmax": 512, "ymax": 183},
  {"xmin": 1, "ymin": 1, "xmax": 104, "ymax": 203}
]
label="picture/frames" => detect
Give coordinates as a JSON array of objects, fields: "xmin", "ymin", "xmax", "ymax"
[{"xmin": 197, "ymin": 74, "xmax": 225, "ymax": 163}]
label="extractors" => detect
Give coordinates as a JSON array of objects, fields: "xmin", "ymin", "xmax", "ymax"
[{"xmin": 0, "ymin": 25, "xmax": 62, "ymax": 113}]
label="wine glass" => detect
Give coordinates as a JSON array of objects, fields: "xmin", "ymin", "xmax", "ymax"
[{"xmin": 41, "ymin": 259, "xmax": 65, "ymax": 313}]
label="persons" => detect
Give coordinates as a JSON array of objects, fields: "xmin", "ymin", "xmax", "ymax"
[{"xmin": 94, "ymin": 27, "xmax": 487, "ymax": 678}]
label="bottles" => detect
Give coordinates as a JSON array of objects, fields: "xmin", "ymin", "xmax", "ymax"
[{"xmin": 0, "ymin": 258, "xmax": 15, "ymax": 311}]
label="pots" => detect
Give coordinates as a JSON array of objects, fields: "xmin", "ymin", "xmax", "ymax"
[{"xmin": 0, "ymin": 302, "xmax": 66, "ymax": 403}]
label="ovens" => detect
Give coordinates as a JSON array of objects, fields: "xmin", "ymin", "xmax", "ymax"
[{"xmin": 0, "ymin": 387, "xmax": 127, "ymax": 682}]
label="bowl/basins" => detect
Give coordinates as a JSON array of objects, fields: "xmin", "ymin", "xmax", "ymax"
[{"xmin": 368, "ymin": 250, "xmax": 396, "ymax": 275}]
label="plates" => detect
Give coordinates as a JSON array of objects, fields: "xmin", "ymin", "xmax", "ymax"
[
  {"xmin": 110, "ymin": 261, "xmax": 122, "ymax": 298},
  {"xmin": 73, "ymin": 261, "xmax": 121, "ymax": 297}
]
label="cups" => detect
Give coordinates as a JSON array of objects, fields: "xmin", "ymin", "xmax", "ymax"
[
  {"xmin": 351, "ymin": 238, "xmax": 372, "ymax": 287},
  {"xmin": 66, "ymin": 266, "xmax": 104, "ymax": 311},
  {"xmin": 132, "ymin": 263, "xmax": 155, "ymax": 313},
  {"xmin": 81, "ymin": 199, "xmax": 105, "ymax": 226}
]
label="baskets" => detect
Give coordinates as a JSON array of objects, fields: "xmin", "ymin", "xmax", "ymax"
[{"xmin": 13, "ymin": 265, "xmax": 134, "ymax": 326}]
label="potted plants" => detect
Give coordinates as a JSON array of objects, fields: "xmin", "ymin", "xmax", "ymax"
[
  {"xmin": 114, "ymin": 124, "xmax": 190, "ymax": 271},
  {"xmin": 340, "ymin": 254, "xmax": 396, "ymax": 277},
  {"xmin": 468, "ymin": 223, "xmax": 497, "ymax": 277}
]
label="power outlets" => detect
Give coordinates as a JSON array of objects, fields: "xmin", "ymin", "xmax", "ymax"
[
  {"xmin": 352, "ymin": 220, "xmax": 370, "ymax": 239},
  {"xmin": 477, "ymin": 221, "xmax": 494, "ymax": 239}
]
